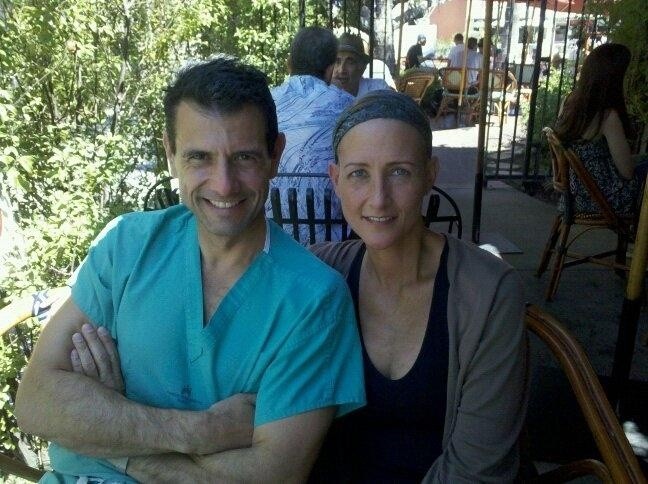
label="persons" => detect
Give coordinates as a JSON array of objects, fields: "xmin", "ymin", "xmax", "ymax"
[
  {"xmin": 12, "ymin": 53, "xmax": 372, "ymax": 484},
  {"xmin": 567, "ymin": 39, "xmax": 586, "ymax": 77},
  {"xmin": 403, "ymin": 32, "xmax": 438, "ymax": 68},
  {"xmin": 326, "ymin": 28, "xmax": 400, "ymax": 102},
  {"xmin": 548, "ymin": 40, "xmax": 647, "ymax": 225},
  {"xmin": 258, "ymin": 23, "xmax": 357, "ymax": 252},
  {"xmin": 443, "ymin": 30, "xmax": 511, "ymax": 119},
  {"xmin": 300, "ymin": 86, "xmax": 536, "ymax": 483}
]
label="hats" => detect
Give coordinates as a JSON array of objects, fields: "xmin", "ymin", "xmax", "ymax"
[{"xmin": 337, "ymin": 32, "xmax": 370, "ymax": 63}]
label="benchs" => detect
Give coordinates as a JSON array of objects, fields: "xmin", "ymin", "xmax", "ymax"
[
  {"xmin": 144, "ymin": 172, "xmax": 463, "ymax": 247},
  {"xmin": 1, "ymin": 286, "xmax": 648, "ymax": 484}
]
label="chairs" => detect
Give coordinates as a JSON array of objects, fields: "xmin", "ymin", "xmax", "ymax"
[
  {"xmin": 535, "ymin": 127, "xmax": 639, "ymax": 302},
  {"xmin": 398, "ymin": 57, "xmax": 532, "ymax": 128}
]
